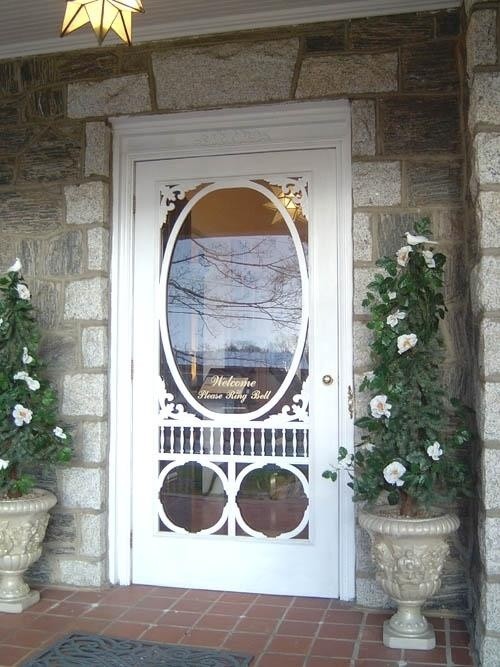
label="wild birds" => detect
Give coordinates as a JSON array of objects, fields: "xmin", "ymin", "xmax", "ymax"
[
  {"xmin": 399, "ymin": 231, "xmax": 440, "ymax": 246},
  {"xmin": 5, "ymin": 257, "xmax": 24, "ymax": 273}
]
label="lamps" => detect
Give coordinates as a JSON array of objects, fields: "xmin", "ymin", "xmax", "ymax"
[
  {"xmin": 263, "ymin": 185, "xmax": 308, "ymax": 228},
  {"xmin": 60, "ymin": 0, "xmax": 143, "ymax": 45}
]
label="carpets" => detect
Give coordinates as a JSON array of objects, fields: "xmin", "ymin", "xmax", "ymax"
[{"xmin": 25, "ymin": 632, "xmax": 254, "ymax": 666}]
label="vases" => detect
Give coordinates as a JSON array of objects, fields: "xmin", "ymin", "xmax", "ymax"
[
  {"xmin": 355, "ymin": 507, "xmax": 461, "ymax": 651},
  {"xmin": 0, "ymin": 490, "xmax": 57, "ymax": 617}
]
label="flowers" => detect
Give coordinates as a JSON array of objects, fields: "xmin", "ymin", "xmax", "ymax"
[
  {"xmin": 321, "ymin": 214, "xmax": 471, "ymax": 517},
  {"xmin": 0, "ymin": 258, "xmax": 79, "ymax": 498}
]
